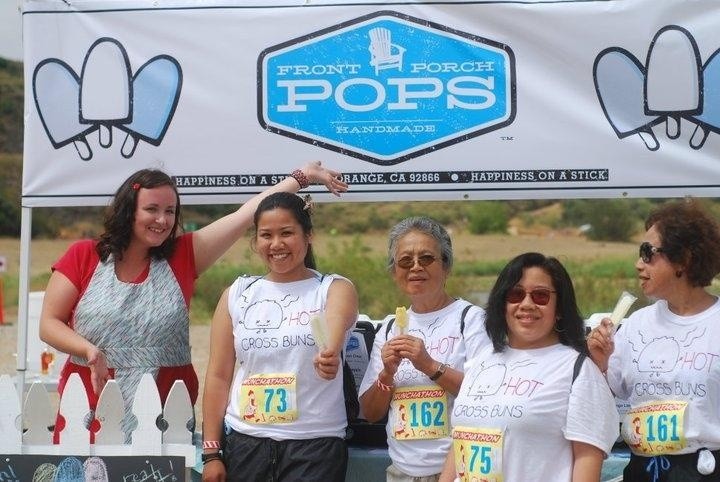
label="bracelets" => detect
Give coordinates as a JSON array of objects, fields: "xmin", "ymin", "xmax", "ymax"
[
  {"xmin": 374, "ymin": 378, "xmax": 395, "ymax": 392},
  {"xmin": 602, "ymin": 369, "xmax": 607, "ymax": 376},
  {"xmin": 202, "ymin": 441, "xmax": 224, "ymax": 465},
  {"xmin": 291, "ymin": 168, "xmax": 310, "ymax": 189}
]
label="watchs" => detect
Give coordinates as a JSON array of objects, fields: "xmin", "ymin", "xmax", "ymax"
[{"xmin": 430, "ymin": 364, "xmax": 447, "ymax": 381}]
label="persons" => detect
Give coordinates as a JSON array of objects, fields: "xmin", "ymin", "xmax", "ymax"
[
  {"xmin": 586, "ymin": 204, "xmax": 720, "ymax": 482},
  {"xmin": 201, "ymin": 193, "xmax": 359, "ymax": 482},
  {"xmin": 358, "ymin": 215, "xmax": 494, "ymax": 482},
  {"xmin": 39, "ymin": 161, "xmax": 349, "ymax": 444},
  {"xmin": 437, "ymin": 253, "xmax": 619, "ymax": 482}
]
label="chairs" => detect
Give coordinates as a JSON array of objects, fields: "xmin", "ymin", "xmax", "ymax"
[
  {"xmin": 368, "ymin": 26, "xmax": 407, "ymax": 75},
  {"xmin": 11, "ymin": 292, "xmax": 73, "ymax": 390}
]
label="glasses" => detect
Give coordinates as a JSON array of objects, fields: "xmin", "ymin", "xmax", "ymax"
[
  {"xmin": 640, "ymin": 243, "xmax": 667, "ymax": 263},
  {"xmin": 506, "ymin": 290, "xmax": 556, "ymax": 305},
  {"xmin": 398, "ymin": 256, "xmax": 444, "ymax": 268}
]
